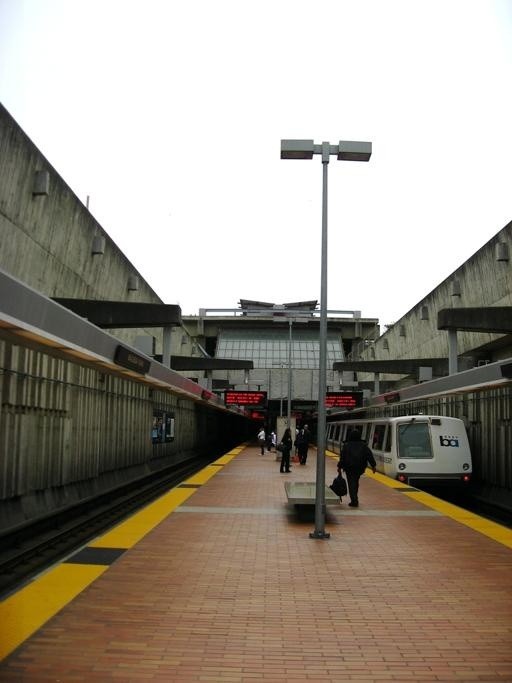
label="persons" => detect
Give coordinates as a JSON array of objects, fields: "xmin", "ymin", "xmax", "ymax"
[
  {"xmin": 336, "ymin": 429, "xmax": 377, "ymax": 507},
  {"xmin": 279, "ymin": 428, "xmax": 293, "ymax": 473},
  {"xmin": 257, "ymin": 427, "xmax": 266, "ymax": 456},
  {"xmin": 267, "ymin": 432, "xmax": 276, "ymax": 452},
  {"xmin": 295, "ymin": 424, "xmax": 312, "ymax": 466}
]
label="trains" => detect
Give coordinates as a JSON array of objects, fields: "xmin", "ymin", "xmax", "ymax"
[{"xmin": 325, "ymin": 414, "xmax": 474, "ymax": 493}]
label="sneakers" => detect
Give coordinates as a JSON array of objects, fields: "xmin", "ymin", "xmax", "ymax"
[{"xmin": 349, "ymin": 503, "xmax": 358, "ymax": 506}]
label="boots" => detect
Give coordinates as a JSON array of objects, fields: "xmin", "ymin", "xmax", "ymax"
[{"xmin": 280, "ymin": 466, "xmax": 290, "ymax": 472}]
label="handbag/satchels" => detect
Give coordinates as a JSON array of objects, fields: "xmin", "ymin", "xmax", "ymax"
[
  {"xmin": 330, "ymin": 478, "xmax": 347, "ymax": 496},
  {"xmin": 278, "ymin": 445, "xmax": 287, "ymax": 451},
  {"xmin": 295, "ymin": 440, "xmax": 305, "ymax": 446}
]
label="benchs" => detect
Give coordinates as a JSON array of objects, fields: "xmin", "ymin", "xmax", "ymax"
[{"xmin": 283, "ymin": 480, "xmax": 340, "ymax": 524}]
